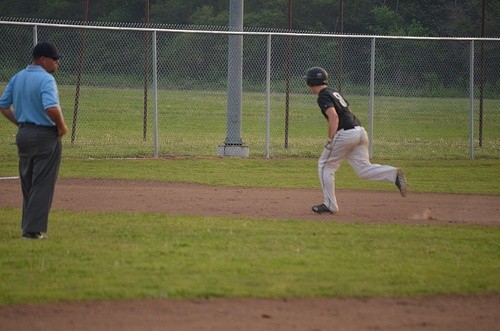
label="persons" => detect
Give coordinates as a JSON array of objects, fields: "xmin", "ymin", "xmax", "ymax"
[
  {"xmin": 0, "ymin": 43, "xmax": 69, "ymax": 241},
  {"xmin": 302, "ymin": 67, "xmax": 408, "ymax": 213}
]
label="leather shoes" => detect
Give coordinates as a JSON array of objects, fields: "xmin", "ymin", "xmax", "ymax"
[{"xmin": 22, "ymin": 232, "xmax": 44, "ymax": 239}]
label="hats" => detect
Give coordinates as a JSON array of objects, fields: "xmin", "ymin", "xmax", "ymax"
[{"xmin": 32, "ymin": 42, "xmax": 62, "ymax": 58}]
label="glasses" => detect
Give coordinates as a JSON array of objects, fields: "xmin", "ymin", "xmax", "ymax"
[{"xmin": 46, "ymin": 56, "xmax": 59, "ymax": 61}]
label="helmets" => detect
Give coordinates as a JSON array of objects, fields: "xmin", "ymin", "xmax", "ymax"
[{"xmin": 302, "ymin": 66, "xmax": 329, "ymax": 79}]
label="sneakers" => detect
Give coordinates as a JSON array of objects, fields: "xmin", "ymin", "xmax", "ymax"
[
  {"xmin": 395, "ymin": 168, "xmax": 407, "ymax": 197},
  {"xmin": 312, "ymin": 204, "xmax": 335, "ymax": 214}
]
